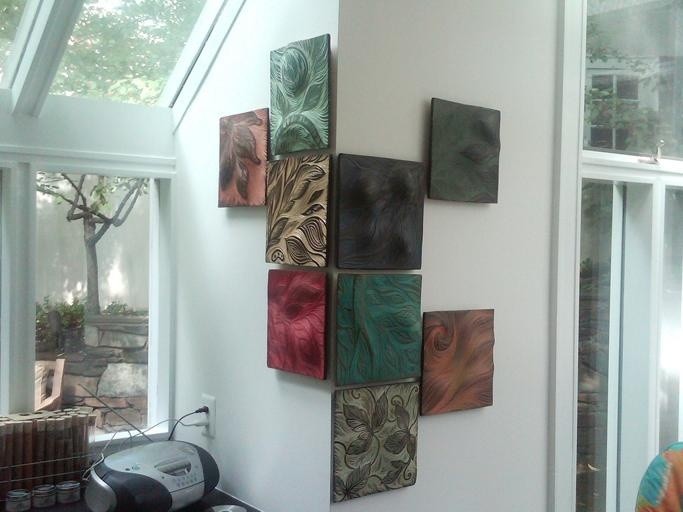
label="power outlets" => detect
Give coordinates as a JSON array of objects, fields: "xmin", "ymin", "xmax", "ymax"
[{"xmin": 202, "ymin": 395, "xmax": 215, "ymax": 439}]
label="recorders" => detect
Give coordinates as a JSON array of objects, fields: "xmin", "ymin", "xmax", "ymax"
[{"xmin": 84, "ymin": 440, "xmax": 220, "ymax": 512}]
